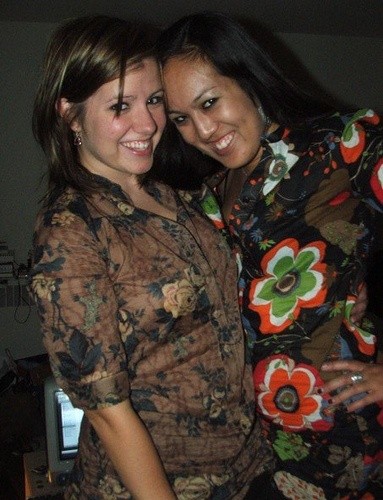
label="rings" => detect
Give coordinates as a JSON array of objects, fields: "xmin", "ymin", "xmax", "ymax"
[{"xmin": 350, "ymin": 371, "xmax": 363, "ymax": 384}]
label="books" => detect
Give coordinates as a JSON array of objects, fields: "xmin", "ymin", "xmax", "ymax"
[{"xmin": 0, "ymin": 241, "xmax": 28, "ymax": 286}]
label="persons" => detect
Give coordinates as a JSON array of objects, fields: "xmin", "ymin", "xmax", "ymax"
[
  {"xmin": 161, "ymin": 7, "xmax": 383, "ymax": 500},
  {"xmin": 26, "ymin": 14, "xmax": 264, "ymax": 500}
]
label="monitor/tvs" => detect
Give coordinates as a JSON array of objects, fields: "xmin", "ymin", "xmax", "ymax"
[{"xmin": 44, "ymin": 375, "xmax": 84, "ymax": 474}]
